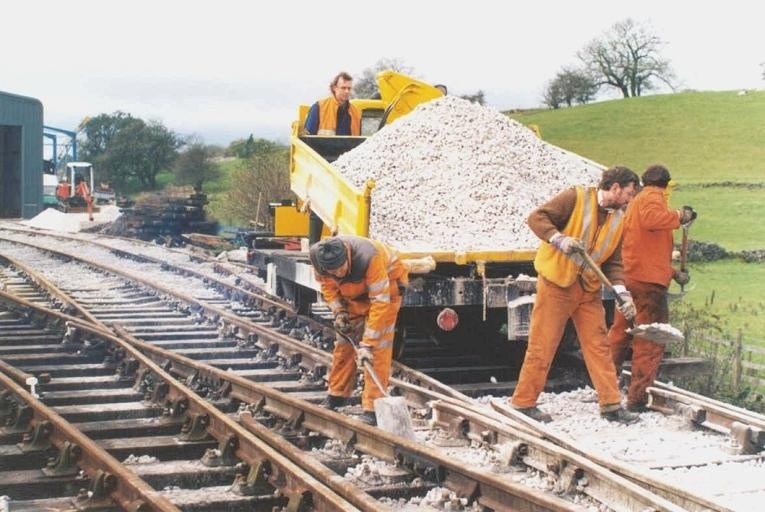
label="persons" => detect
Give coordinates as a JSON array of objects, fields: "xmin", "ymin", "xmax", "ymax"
[
  {"xmin": 303, "ymin": 73, "xmax": 363, "ymax": 243},
  {"xmin": 310, "ymin": 235, "xmax": 409, "ymax": 425},
  {"xmin": 609, "ymin": 166, "xmax": 696, "ymax": 412},
  {"xmin": 511, "ymin": 166, "xmax": 643, "ymax": 424}
]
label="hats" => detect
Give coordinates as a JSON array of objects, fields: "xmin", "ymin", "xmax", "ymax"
[{"xmin": 316, "ymin": 239, "xmax": 345, "ymax": 268}]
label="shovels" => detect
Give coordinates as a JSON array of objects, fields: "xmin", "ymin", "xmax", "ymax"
[
  {"xmin": 572, "ymin": 241, "xmax": 685, "ymax": 343},
  {"xmin": 335, "ymin": 325, "xmax": 416, "ymax": 441}
]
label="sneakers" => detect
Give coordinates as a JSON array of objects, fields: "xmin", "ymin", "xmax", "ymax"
[
  {"xmin": 321, "ymin": 395, "xmax": 376, "ymax": 424},
  {"xmin": 602, "ymin": 409, "xmax": 639, "ymax": 424},
  {"xmin": 515, "ymin": 406, "xmax": 551, "ymax": 422}
]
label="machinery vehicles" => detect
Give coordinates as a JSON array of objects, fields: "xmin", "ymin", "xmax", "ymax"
[
  {"xmin": 56, "ymin": 161, "xmax": 101, "ymax": 214},
  {"xmin": 240, "ymin": 71, "xmax": 666, "ymax": 363}
]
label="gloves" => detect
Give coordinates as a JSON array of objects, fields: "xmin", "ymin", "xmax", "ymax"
[
  {"xmin": 675, "ymin": 271, "xmax": 689, "ymax": 283},
  {"xmin": 680, "ymin": 207, "xmax": 696, "ymax": 223},
  {"xmin": 335, "ymin": 312, "xmax": 352, "ymax": 334},
  {"xmin": 616, "ymin": 290, "xmax": 637, "ymax": 320},
  {"xmin": 357, "ymin": 348, "xmax": 374, "ymax": 370},
  {"xmin": 561, "ymin": 237, "xmax": 585, "ymax": 255}
]
